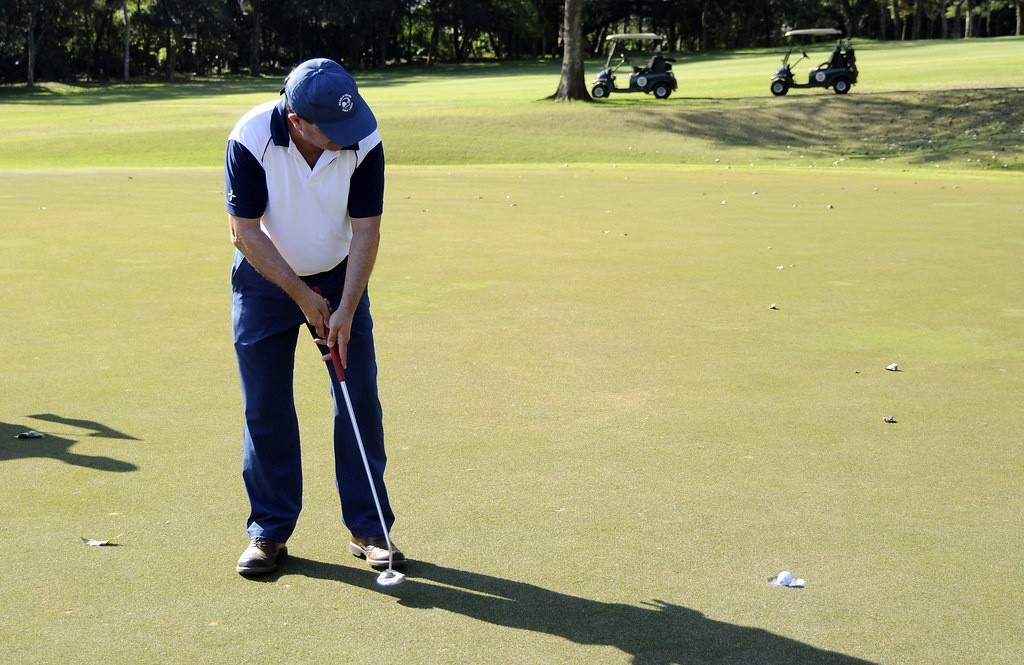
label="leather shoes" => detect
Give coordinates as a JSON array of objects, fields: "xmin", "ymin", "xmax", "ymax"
[
  {"xmin": 349, "ymin": 534, "xmax": 407, "ymax": 568},
  {"xmin": 237, "ymin": 537, "xmax": 288, "ymax": 576}
]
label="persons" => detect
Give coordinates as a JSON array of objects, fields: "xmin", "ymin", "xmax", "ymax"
[{"xmin": 222, "ymin": 58, "xmax": 410, "ymax": 573}]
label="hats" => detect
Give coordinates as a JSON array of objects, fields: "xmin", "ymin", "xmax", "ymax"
[{"xmin": 280, "ymin": 58, "xmax": 377, "ymax": 147}]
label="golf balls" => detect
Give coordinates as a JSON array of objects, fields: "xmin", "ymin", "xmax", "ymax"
[
  {"xmin": 777, "ymin": 570, "xmax": 793, "ymax": 586},
  {"xmin": 324, "ymin": 321, "xmax": 408, "ymax": 587}
]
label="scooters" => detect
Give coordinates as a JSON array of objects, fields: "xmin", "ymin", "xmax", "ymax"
[
  {"xmin": 771, "ymin": 28, "xmax": 858, "ymax": 96},
  {"xmin": 591, "ymin": 33, "xmax": 678, "ymax": 99}
]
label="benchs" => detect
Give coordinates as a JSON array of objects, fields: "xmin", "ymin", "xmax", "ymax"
[
  {"xmin": 809, "ymin": 45, "xmax": 841, "ymax": 73},
  {"xmin": 632, "ymin": 55, "xmax": 657, "ymax": 73}
]
